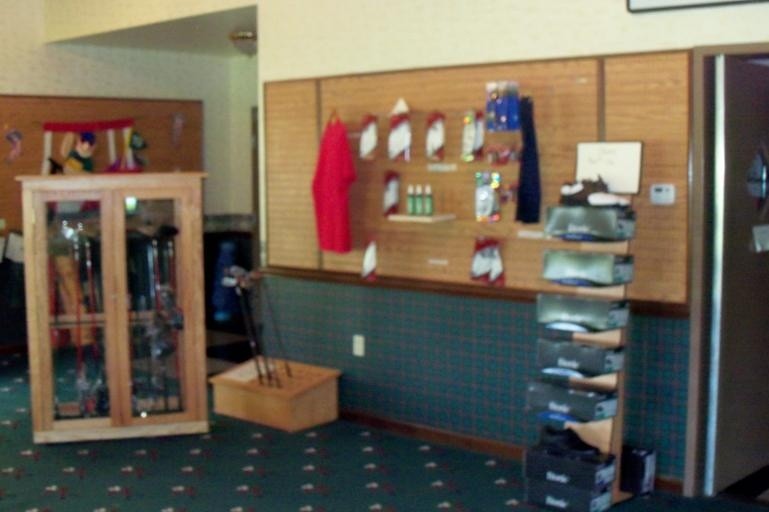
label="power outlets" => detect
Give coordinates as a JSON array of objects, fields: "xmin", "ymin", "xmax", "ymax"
[{"xmin": 353, "ymin": 334, "xmax": 366, "ymax": 358}]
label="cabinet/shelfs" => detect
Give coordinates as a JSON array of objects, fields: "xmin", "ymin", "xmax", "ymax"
[{"xmin": 16, "ymin": 173, "xmax": 210, "ymax": 443}]
